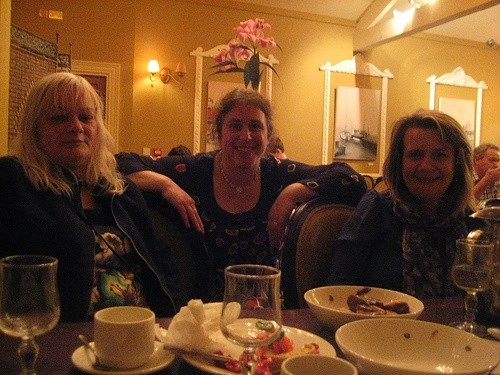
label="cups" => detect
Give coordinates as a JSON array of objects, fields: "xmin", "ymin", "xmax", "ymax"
[
  {"xmin": 94, "ymin": 306, "xmax": 155, "ymax": 369},
  {"xmin": 281, "ymin": 356, "xmax": 358, "ymax": 375}
]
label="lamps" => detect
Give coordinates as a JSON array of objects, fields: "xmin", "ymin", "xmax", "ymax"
[{"xmin": 146, "ymin": 60, "xmax": 188, "ymax": 91}]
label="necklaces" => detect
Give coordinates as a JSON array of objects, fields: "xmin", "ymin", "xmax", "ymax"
[{"xmin": 219, "ymin": 152, "xmax": 261, "ymax": 193}]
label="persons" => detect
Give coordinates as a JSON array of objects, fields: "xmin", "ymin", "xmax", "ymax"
[
  {"xmin": 472, "ymin": 143, "xmax": 500, "ymax": 200},
  {"xmin": 168, "ymin": 145, "xmax": 192, "ymax": 156},
  {"xmin": 118, "ymin": 87, "xmax": 367, "ymax": 310},
  {"xmin": 0, "ymin": 72, "xmax": 188, "ymax": 322},
  {"xmin": 269, "ymin": 137, "xmax": 288, "ymax": 159},
  {"xmin": 322, "ymin": 109, "xmax": 480, "ymax": 299}
]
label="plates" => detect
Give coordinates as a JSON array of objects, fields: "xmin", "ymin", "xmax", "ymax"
[
  {"xmin": 181, "ymin": 318, "xmax": 336, "ymax": 375},
  {"xmin": 71, "ymin": 342, "xmax": 177, "ymax": 375}
]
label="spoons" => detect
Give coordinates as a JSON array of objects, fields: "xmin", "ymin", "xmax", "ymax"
[{"xmin": 79, "ymin": 334, "xmax": 117, "ymax": 371}]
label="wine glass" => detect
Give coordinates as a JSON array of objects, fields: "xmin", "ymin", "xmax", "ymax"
[
  {"xmin": 0, "ymin": 255, "xmax": 60, "ymax": 375},
  {"xmin": 448, "ymin": 239, "xmax": 494, "ymax": 337},
  {"xmin": 220, "ymin": 264, "xmax": 282, "ymax": 375}
]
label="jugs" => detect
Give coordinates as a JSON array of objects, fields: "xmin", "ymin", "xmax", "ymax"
[{"xmin": 469, "ymin": 198, "xmax": 500, "ymax": 313}]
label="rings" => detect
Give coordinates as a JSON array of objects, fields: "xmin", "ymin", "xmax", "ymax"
[{"xmin": 193, "ymin": 209, "xmax": 196, "ymax": 210}]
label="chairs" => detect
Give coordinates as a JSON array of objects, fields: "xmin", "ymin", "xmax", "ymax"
[{"xmin": 283, "ymin": 195, "xmax": 358, "ymax": 309}]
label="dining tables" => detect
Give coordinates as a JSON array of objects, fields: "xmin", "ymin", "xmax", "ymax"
[{"xmin": 0, "ymin": 296, "xmax": 500, "ymax": 375}]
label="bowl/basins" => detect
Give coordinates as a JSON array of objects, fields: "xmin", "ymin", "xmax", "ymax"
[
  {"xmin": 304, "ymin": 286, "xmax": 424, "ymax": 331},
  {"xmin": 336, "ymin": 318, "xmax": 500, "ymax": 375}
]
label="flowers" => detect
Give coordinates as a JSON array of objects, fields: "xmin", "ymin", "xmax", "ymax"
[{"xmin": 208, "ymin": 18, "xmax": 282, "ymax": 90}]
